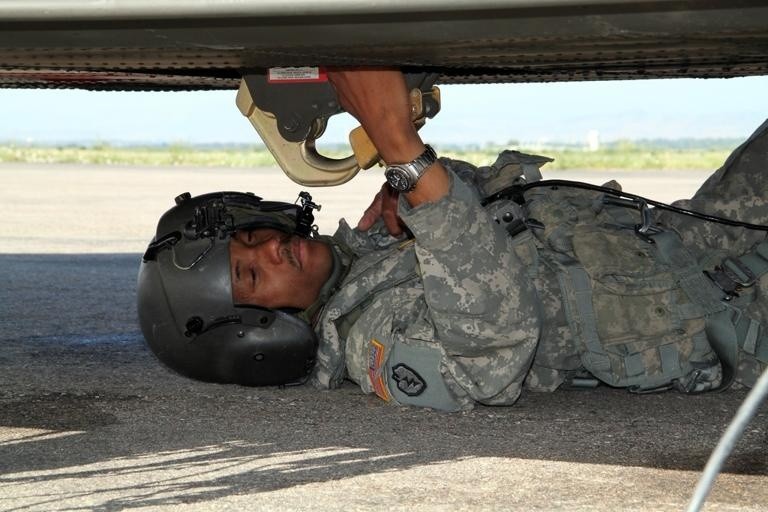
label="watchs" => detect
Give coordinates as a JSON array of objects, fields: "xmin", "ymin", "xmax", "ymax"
[{"xmin": 382, "ymin": 143, "xmax": 435, "ymax": 194}]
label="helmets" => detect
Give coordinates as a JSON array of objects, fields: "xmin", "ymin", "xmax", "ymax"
[{"xmin": 135, "ymin": 190, "xmax": 320, "ymax": 390}]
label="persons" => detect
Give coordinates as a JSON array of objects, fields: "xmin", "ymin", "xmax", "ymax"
[{"xmin": 135, "ymin": 68, "xmax": 768, "ymax": 402}]
